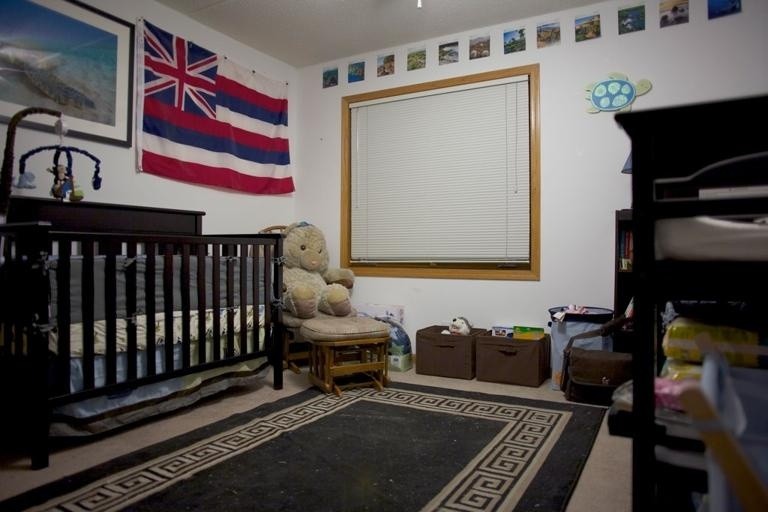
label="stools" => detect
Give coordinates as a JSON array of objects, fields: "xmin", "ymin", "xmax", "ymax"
[{"xmin": 302, "ymin": 318, "xmax": 392, "ymax": 397}]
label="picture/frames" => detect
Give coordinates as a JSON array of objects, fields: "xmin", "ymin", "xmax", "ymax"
[{"xmin": 0, "ymin": 0, "xmax": 135, "ymax": 146}]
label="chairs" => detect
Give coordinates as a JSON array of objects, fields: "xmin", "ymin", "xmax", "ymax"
[{"xmin": 258, "ymin": 225, "xmax": 355, "ymax": 373}]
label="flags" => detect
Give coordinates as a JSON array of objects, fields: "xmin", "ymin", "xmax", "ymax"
[{"xmin": 137, "ymin": 17, "xmax": 295, "ymax": 195}]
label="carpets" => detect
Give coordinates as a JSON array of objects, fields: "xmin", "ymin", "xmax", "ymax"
[{"xmin": 0, "ymin": 378, "xmax": 609, "ymax": 509}]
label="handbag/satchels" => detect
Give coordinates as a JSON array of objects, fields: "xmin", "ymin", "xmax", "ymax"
[{"xmin": 560, "ymin": 329, "xmax": 633, "ymax": 405}]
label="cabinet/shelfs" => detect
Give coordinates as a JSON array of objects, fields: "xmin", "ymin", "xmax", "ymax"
[{"xmin": 614, "ymin": 96, "xmax": 767, "ymax": 512}]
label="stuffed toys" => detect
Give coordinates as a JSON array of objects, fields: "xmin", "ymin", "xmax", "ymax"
[
  {"xmin": 11, "ymin": 145, "xmax": 102, "ymax": 201},
  {"xmin": 282, "ymin": 222, "xmax": 354, "ymax": 319},
  {"xmin": 441, "ymin": 316, "xmax": 472, "ymax": 336}
]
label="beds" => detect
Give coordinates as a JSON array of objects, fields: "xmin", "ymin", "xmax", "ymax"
[{"xmin": 0, "ymin": 193, "xmax": 285, "ymax": 469}]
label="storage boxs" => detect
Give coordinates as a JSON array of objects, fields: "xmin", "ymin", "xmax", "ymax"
[
  {"xmin": 549, "ymin": 306, "xmax": 612, "ymax": 390},
  {"xmin": 475, "ymin": 329, "xmax": 551, "ymax": 386},
  {"xmin": 414, "ymin": 325, "xmax": 486, "ymax": 379}
]
